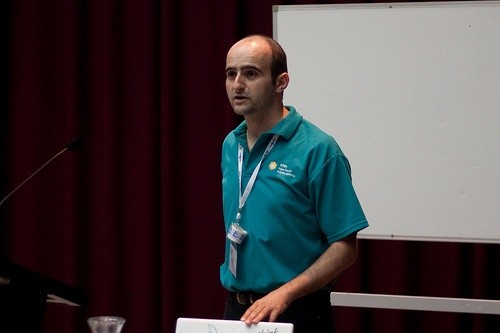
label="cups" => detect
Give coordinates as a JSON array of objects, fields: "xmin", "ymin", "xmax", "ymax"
[{"xmin": 88, "ymin": 316, "xmax": 126, "ymax": 333}]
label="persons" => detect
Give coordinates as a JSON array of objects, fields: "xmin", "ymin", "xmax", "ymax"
[{"xmin": 220, "ymin": 36, "xmax": 369, "ymax": 333}]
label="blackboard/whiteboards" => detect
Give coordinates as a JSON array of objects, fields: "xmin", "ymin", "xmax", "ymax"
[{"xmin": 272, "ymin": 0, "xmax": 500, "ymax": 245}]
